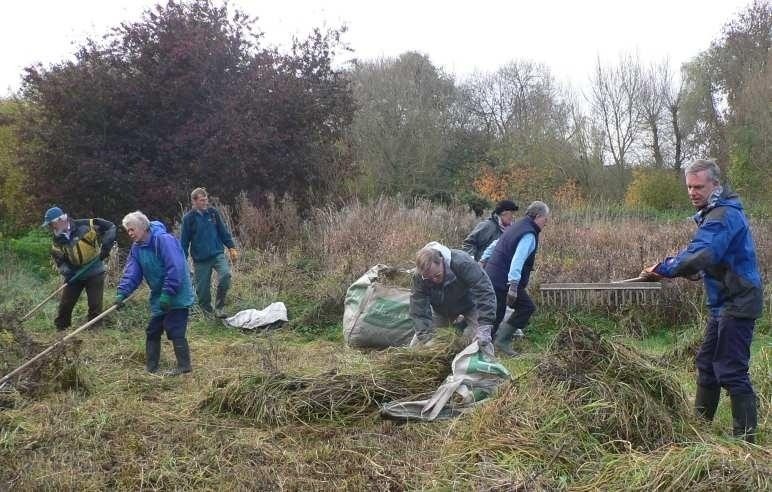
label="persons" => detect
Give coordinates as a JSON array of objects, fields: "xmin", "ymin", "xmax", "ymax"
[
  {"xmin": 462, "ymin": 200, "xmax": 519, "ymax": 263},
  {"xmin": 478, "ymin": 201, "xmax": 550, "ymax": 356},
  {"xmin": 181, "ymin": 188, "xmax": 238, "ymax": 320},
  {"xmin": 111, "ymin": 210, "xmax": 197, "ymax": 376},
  {"xmin": 41, "ymin": 206, "xmax": 117, "ymax": 332},
  {"xmin": 638, "ymin": 158, "xmax": 764, "ymax": 444},
  {"xmin": 408, "ymin": 241, "xmax": 497, "ymax": 351}
]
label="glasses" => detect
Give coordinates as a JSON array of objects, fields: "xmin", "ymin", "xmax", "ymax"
[{"xmin": 422, "ymin": 271, "xmax": 443, "ymax": 281}]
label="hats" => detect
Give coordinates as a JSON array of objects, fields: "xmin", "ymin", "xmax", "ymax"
[
  {"xmin": 495, "ymin": 199, "xmax": 519, "ymax": 214},
  {"xmin": 41, "ymin": 207, "xmax": 65, "ymax": 227}
]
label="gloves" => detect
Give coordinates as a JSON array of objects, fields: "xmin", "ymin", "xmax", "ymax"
[
  {"xmin": 99, "ymin": 248, "xmax": 110, "ymax": 261},
  {"xmin": 160, "ymin": 293, "xmax": 172, "ymax": 312},
  {"xmin": 638, "ymin": 263, "xmax": 664, "ymax": 282},
  {"xmin": 506, "ymin": 283, "xmax": 518, "ymax": 306},
  {"xmin": 472, "ymin": 325, "xmax": 492, "ymax": 346},
  {"xmin": 64, "ymin": 274, "xmax": 78, "ymax": 287},
  {"xmin": 112, "ymin": 294, "xmax": 125, "ymax": 310},
  {"xmin": 230, "ymin": 248, "xmax": 238, "ymax": 263}
]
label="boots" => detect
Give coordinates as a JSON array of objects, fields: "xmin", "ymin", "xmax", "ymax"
[
  {"xmin": 146, "ymin": 339, "xmax": 160, "ymax": 373},
  {"xmin": 727, "ymin": 391, "xmax": 757, "ymax": 443},
  {"xmin": 166, "ymin": 338, "xmax": 192, "ymax": 377},
  {"xmin": 199, "ymin": 286, "xmax": 227, "ymax": 318},
  {"xmin": 693, "ymin": 384, "xmax": 720, "ymax": 424},
  {"xmin": 494, "ymin": 321, "xmax": 520, "ymax": 355}
]
